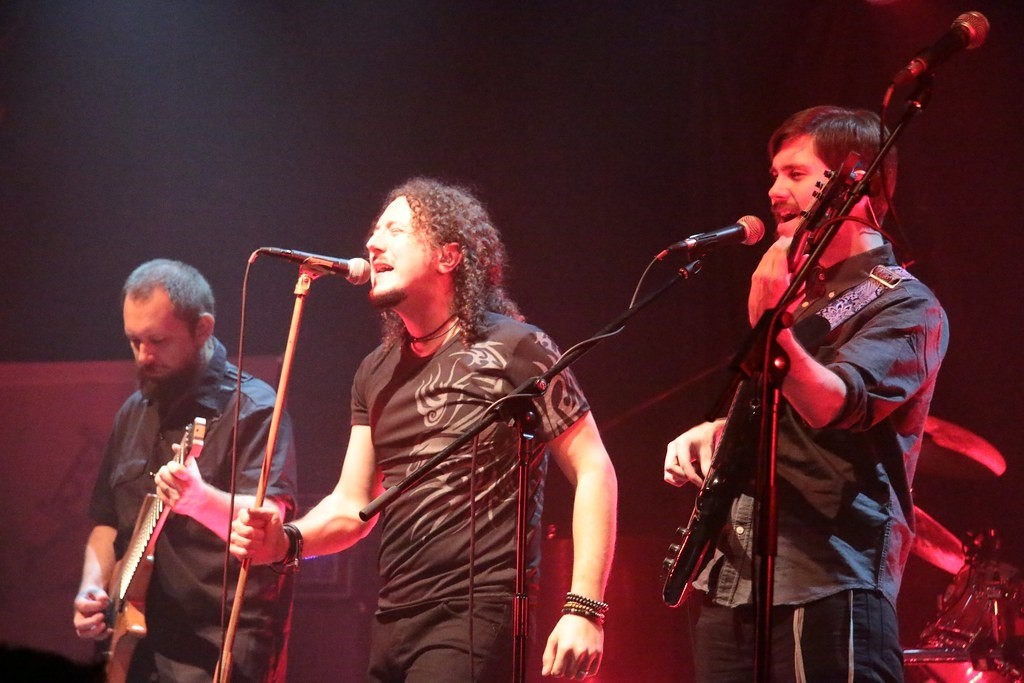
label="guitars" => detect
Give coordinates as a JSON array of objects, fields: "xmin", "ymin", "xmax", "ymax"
[
  {"xmin": 98, "ymin": 418, "xmax": 208, "ymax": 682},
  {"xmin": 661, "ymin": 150, "xmax": 864, "ymax": 608}
]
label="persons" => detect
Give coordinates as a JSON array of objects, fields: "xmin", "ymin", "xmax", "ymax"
[
  {"xmin": 229, "ymin": 179, "xmax": 617, "ymax": 683},
  {"xmin": 73, "ymin": 259, "xmax": 300, "ymax": 683},
  {"xmin": 664, "ymin": 108, "xmax": 950, "ymax": 683}
]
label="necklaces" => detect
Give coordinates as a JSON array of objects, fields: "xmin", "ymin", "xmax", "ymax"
[{"xmin": 410, "ymin": 312, "xmax": 461, "ymax": 342}]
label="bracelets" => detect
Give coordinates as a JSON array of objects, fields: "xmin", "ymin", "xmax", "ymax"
[
  {"xmin": 561, "ymin": 592, "xmax": 609, "ymax": 622},
  {"xmin": 268, "ymin": 524, "xmax": 304, "ymax": 575}
]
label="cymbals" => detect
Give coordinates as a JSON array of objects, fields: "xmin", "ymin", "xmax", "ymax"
[
  {"xmin": 910, "ymin": 503, "xmax": 970, "ymax": 574},
  {"xmin": 914, "ymin": 413, "xmax": 1008, "ymax": 480}
]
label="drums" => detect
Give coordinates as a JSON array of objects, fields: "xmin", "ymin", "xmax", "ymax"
[{"xmin": 913, "ymin": 532, "xmax": 1024, "ymax": 683}]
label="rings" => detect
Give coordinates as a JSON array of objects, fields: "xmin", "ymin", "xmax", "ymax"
[
  {"xmin": 579, "ymin": 670, "xmax": 588, "ymax": 675},
  {"xmin": 165, "ymin": 487, "xmax": 169, "ymax": 493}
]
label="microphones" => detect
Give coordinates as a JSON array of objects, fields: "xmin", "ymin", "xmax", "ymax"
[
  {"xmin": 892, "ymin": 11, "xmax": 989, "ymax": 89},
  {"xmin": 259, "ymin": 247, "xmax": 371, "ymax": 285},
  {"xmin": 667, "ymin": 215, "xmax": 766, "ymax": 253}
]
